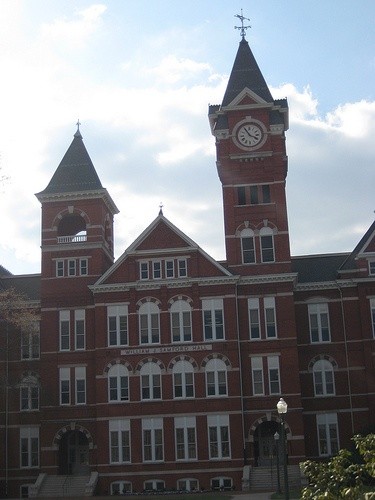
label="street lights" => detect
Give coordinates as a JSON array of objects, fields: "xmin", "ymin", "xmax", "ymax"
[
  {"xmin": 277, "ymin": 397, "xmax": 290, "ymax": 499},
  {"xmin": 274, "ymin": 432, "xmax": 281, "ymax": 492}
]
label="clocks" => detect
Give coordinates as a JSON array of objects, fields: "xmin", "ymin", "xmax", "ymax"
[{"xmin": 238, "ymin": 125, "xmax": 261, "ymax": 146}]
